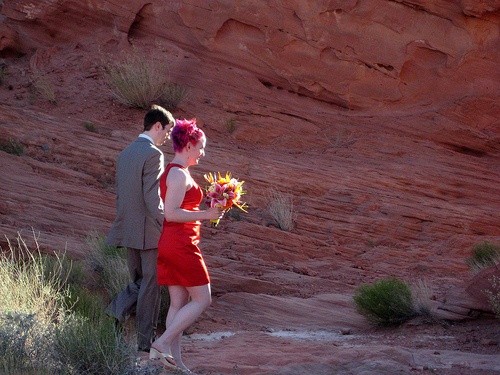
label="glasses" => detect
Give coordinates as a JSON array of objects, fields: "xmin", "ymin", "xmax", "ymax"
[{"xmin": 161, "ymin": 124, "xmax": 172, "ymax": 134}]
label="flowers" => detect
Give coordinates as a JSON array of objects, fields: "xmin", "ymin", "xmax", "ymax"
[{"xmin": 200, "ymin": 170, "xmax": 250, "ymax": 229}]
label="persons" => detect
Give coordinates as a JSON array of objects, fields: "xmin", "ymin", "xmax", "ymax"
[
  {"xmin": 103, "ymin": 104, "xmax": 177, "ymax": 353},
  {"xmin": 149, "ymin": 118, "xmax": 227, "ymax": 375}
]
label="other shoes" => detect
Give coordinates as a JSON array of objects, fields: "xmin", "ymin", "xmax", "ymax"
[
  {"xmin": 108, "ymin": 316, "xmax": 128, "ymax": 337},
  {"xmin": 139, "ymin": 344, "xmax": 153, "ymax": 352}
]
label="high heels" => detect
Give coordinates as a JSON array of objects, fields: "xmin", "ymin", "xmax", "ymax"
[
  {"xmin": 175, "ymin": 361, "xmax": 197, "ymax": 375},
  {"xmin": 149, "ymin": 343, "xmax": 178, "ymax": 368}
]
